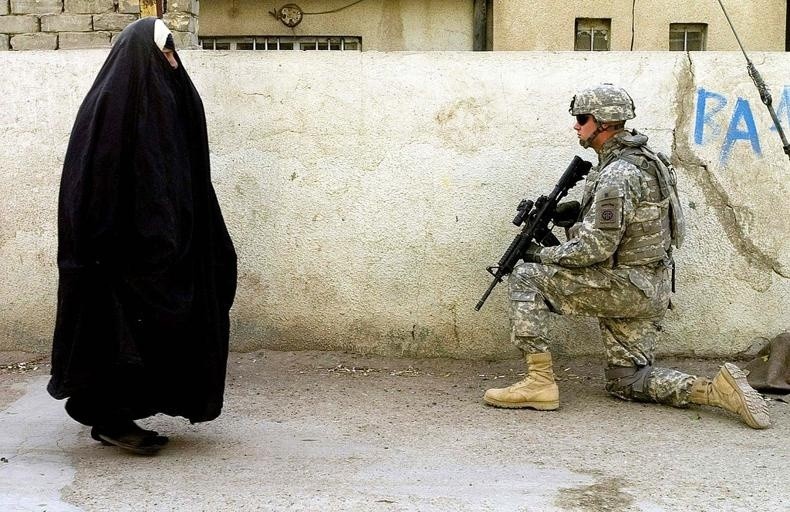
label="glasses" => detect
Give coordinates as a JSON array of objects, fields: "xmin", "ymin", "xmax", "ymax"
[{"xmin": 576, "ymin": 114, "xmax": 592, "ymax": 127}]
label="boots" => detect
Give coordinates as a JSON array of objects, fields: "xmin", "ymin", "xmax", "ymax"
[
  {"xmin": 482, "ymin": 350, "xmax": 560, "ymax": 411},
  {"xmin": 689, "ymin": 362, "xmax": 771, "ymax": 429}
]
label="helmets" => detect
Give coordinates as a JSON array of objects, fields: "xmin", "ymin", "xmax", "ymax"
[{"xmin": 568, "ymin": 82, "xmax": 637, "ymax": 124}]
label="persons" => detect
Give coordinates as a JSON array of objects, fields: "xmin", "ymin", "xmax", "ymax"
[
  {"xmin": 479, "ymin": 81, "xmax": 774, "ymax": 431},
  {"xmin": 41, "ymin": 15, "xmax": 242, "ymax": 459}
]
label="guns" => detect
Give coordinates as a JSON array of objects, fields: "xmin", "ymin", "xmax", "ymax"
[{"xmin": 475, "ymin": 156, "xmax": 593, "ymax": 312}]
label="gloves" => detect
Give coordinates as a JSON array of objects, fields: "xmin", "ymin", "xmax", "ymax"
[{"xmin": 522, "ymin": 241, "xmax": 543, "ymax": 264}]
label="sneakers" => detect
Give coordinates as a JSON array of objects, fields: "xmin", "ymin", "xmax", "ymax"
[{"xmin": 92, "ymin": 421, "xmax": 169, "ymax": 452}]
